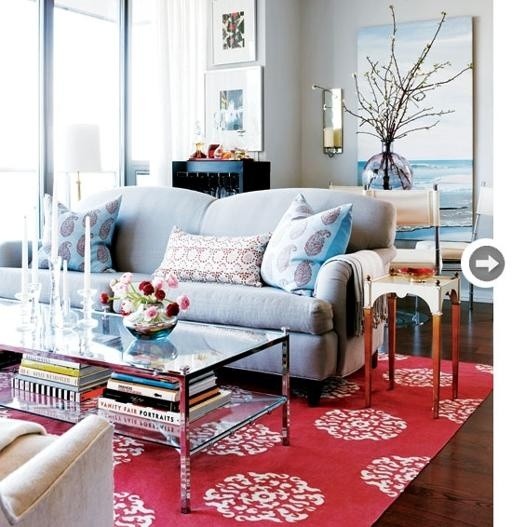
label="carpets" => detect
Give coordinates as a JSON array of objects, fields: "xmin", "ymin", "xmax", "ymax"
[{"xmin": 0, "ymin": 348, "xmax": 493, "ymax": 524}]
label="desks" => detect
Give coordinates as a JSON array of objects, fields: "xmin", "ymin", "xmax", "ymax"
[
  {"xmin": 363, "ymin": 272, "xmax": 460, "ymax": 418},
  {"xmin": 2, "ymin": 296, "xmax": 289, "ymax": 475}
]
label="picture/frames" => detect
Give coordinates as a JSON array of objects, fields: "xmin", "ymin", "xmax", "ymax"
[
  {"xmin": 206, "ymin": 0, "xmax": 257, "ymax": 65},
  {"xmin": 353, "ymin": 17, "xmax": 479, "ymax": 244},
  {"xmin": 203, "ymin": 65, "xmax": 264, "ymax": 149}
]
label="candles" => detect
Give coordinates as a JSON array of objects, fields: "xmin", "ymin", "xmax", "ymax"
[
  {"xmin": 334, "ymin": 129, "xmax": 342, "ymax": 154},
  {"xmin": 22, "ymin": 214, "xmax": 30, "ymax": 284},
  {"xmin": 53, "ymin": 190, "xmax": 62, "ymax": 255},
  {"xmin": 83, "ymin": 214, "xmax": 90, "ymax": 289},
  {"xmin": 323, "ymin": 128, "xmax": 335, "ymax": 157}
]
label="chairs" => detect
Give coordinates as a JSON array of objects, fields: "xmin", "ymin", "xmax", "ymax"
[
  {"xmin": 413, "ymin": 182, "xmax": 493, "ymax": 325},
  {"xmin": 363, "ymin": 182, "xmax": 439, "ymax": 272}
]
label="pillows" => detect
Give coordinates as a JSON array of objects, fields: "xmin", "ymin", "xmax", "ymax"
[
  {"xmin": 35, "ymin": 192, "xmax": 124, "ymax": 271},
  {"xmin": 158, "ymin": 226, "xmax": 267, "ymax": 291},
  {"xmin": 259, "ymin": 194, "xmax": 361, "ymax": 294}
]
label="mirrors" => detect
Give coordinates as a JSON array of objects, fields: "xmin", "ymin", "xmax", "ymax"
[{"xmin": 322, "ymin": 88, "xmax": 344, "ymax": 156}]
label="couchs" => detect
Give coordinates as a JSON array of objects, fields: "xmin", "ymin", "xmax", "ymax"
[
  {"xmin": 0, "ymin": 184, "xmax": 399, "ymax": 408},
  {"xmin": 0, "ymin": 412, "xmax": 117, "ymax": 524}
]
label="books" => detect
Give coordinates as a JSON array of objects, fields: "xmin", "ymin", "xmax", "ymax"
[{"xmin": 11, "ymin": 353, "xmax": 231, "ymax": 425}]
